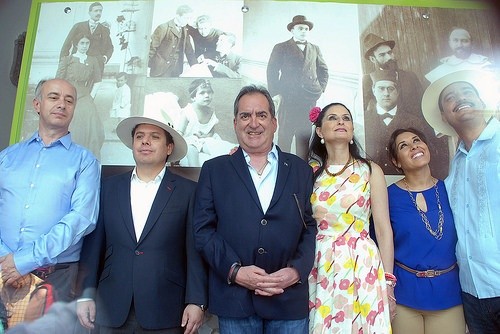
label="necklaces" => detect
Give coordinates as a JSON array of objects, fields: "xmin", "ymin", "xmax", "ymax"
[
  {"xmin": 324, "ymin": 152, "xmax": 351, "ymax": 176},
  {"xmin": 257, "ymin": 160, "xmax": 267, "ymax": 175},
  {"xmin": 403, "ymin": 177, "xmax": 444, "ymax": 240}
]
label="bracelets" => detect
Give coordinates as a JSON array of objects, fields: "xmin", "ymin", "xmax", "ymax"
[
  {"xmin": 385, "ymin": 272, "xmax": 397, "ymax": 287},
  {"xmin": 389, "ymin": 294, "xmax": 396, "ymax": 303},
  {"xmin": 231, "ymin": 265, "xmax": 240, "ymax": 284}
]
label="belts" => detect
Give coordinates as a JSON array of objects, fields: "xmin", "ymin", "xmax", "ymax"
[
  {"xmin": 395, "ymin": 261, "xmax": 457, "ymax": 278},
  {"xmin": 30, "ymin": 262, "xmax": 70, "ymax": 279}
]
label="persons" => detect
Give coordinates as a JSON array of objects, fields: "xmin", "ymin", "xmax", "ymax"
[
  {"xmin": 369, "ymin": 127, "xmax": 466, "ymax": 334},
  {"xmin": 76, "ymin": 109, "xmax": 208, "ymax": 334},
  {"xmin": 0, "ymin": 78, "xmax": 100, "ymax": 334},
  {"xmin": 421, "ymin": 69, "xmax": 500, "ymax": 334},
  {"xmin": 193, "ymin": 84, "xmax": 318, "ymax": 334},
  {"xmin": 306, "ymin": 103, "xmax": 397, "ymax": 334},
  {"xmin": 53, "ymin": 2, "xmax": 500, "ymax": 176}
]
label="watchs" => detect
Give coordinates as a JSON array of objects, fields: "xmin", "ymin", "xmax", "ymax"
[{"xmin": 195, "ymin": 303, "xmax": 207, "ymax": 312}]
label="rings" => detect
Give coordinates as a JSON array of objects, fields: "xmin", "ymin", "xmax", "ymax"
[{"xmin": 392, "ymin": 313, "xmax": 397, "ymax": 318}]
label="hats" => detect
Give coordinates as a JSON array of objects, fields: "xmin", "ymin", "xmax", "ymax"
[
  {"xmin": 369, "ymin": 69, "xmax": 397, "ymax": 86},
  {"xmin": 362, "ymin": 33, "xmax": 396, "ymax": 61},
  {"xmin": 116, "ymin": 114, "xmax": 187, "ymax": 163},
  {"xmin": 287, "ymin": 15, "xmax": 313, "ymax": 32},
  {"xmin": 421, "ymin": 70, "xmax": 498, "ymax": 136}
]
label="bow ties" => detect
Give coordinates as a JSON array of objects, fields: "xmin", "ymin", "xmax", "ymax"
[
  {"xmin": 295, "ymin": 41, "xmax": 308, "ymax": 45},
  {"xmin": 378, "ymin": 113, "xmax": 394, "ymax": 120}
]
